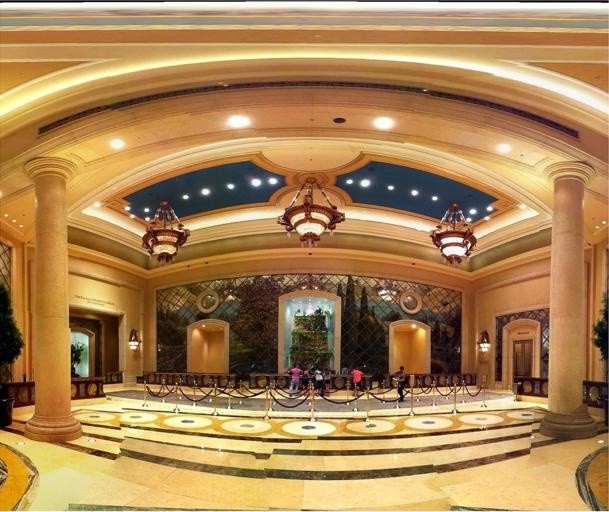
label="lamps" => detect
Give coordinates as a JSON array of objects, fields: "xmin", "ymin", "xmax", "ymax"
[
  {"xmin": 128, "ymin": 328, "xmax": 141, "ymax": 351},
  {"xmin": 430, "ymin": 204, "xmax": 477, "ymax": 268},
  {"xmin": 142, "ymin": 200, "xmax": 191, "ymax": 267},
  {"xmin": 479, "ymin": 330, "xmax": 491, "ymax": 352},
  {"xmin": 276, "ymin": 177, "xmax": 346, "ymax": 250}
]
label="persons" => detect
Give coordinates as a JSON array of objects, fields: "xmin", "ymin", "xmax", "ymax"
[
  {"xmin": 363, "ymin": 364, "xmax": 369, "ymax": 373},
  {"xmin": 351, "ymin": 367, "xmax": 365, "ymax": 392},
  {"xmin": 314, "ymin": 368, "xmax": 323, "ymax": 397},
  {"xmin": 288, "ymin": 362, "xmax": 310, "ymax": 398},
  {"xmin": 392, "ymin": 366, "xmax": 406, "ymax": 402},
  {"xmin": 302, "ymin": 368, "xmax": 311, "ymax": 391},
  {"xmin": 341, "ymin": 365, "xmax": 350, "ymax": 375},
  {"xmin": 322, "ymin": 368, "xmax": 332, "ymax": 391}
]
